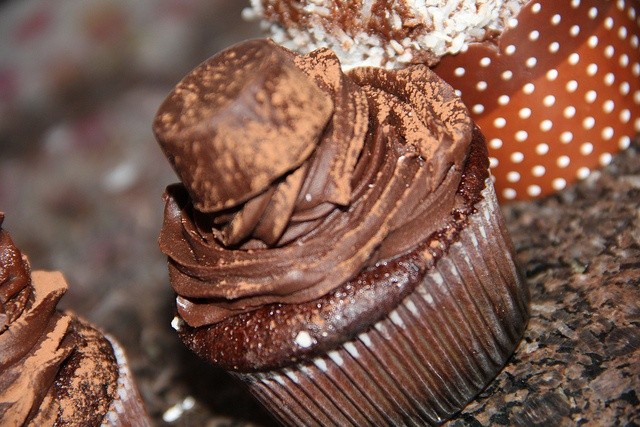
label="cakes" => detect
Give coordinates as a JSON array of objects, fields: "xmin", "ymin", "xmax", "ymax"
[
  {"xmin": 237, "ymin": 1, "xmax": 637, "ymax": 203},
  {"xmin": 0, "ymin": 211, "xmax": 147, "ymax": 426},
  {"xmin": 150, "ymin": 37, "xmax": 530, "ymax": 426}
]
